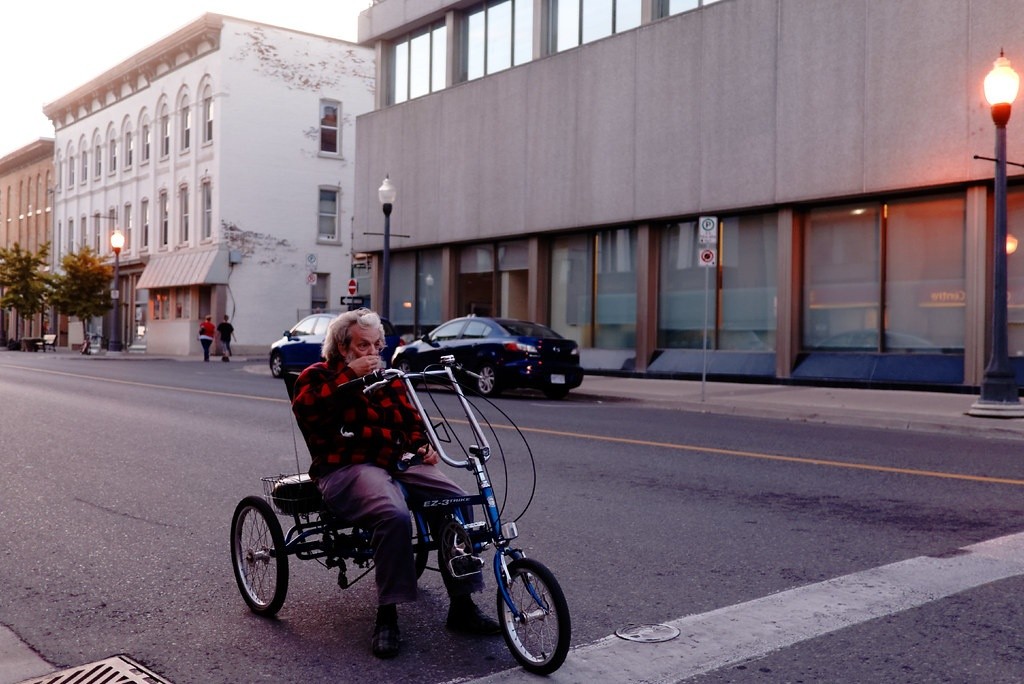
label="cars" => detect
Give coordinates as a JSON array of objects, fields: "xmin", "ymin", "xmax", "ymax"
[
  {"xmin": 391, "ymin": 312, "xmax": 585, "ymax": 402},
  {"xmin": 267, "ymin": 311, "xmax": 405, "ymax": 380}
]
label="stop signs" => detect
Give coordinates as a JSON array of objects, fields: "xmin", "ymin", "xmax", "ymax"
[{"xmin": 349, "ymin": 279, "xmax": 358, "ymax": 294}]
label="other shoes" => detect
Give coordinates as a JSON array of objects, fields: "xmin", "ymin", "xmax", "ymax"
[
  {"xmin": 445, "ymin": 606, "xmax": 501, "ymax": 633},
  {"xmin": 222, "ymin": 357, "xmax": 229, "ymax": 362},
  {"xmin": 372, "ymin": 617, "xmax": 399, "ymax": 657}
]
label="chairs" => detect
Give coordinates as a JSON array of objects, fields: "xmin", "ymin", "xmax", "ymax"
[{"xmin": 515, "ymin": 326, "xmax": 542, "ymax": 336}]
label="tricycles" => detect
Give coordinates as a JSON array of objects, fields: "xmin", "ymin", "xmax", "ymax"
[{"xmin": 229, "ymin": 351, "xmax": 570, "ymax": 677}]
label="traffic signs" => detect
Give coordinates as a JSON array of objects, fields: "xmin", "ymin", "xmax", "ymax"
[{"xmin": 340, "ymin": 296, "xmax": 365, "ymax": 306}]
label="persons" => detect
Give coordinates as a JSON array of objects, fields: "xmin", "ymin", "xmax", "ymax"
[
  {"xmin": 293, "ymin": 307, "xmax": 502, "ymax": 660},
  {"xmin": 216, "ymin": 315, "xmax": 236, "ymax": 362},
  {"xmin": 197, "ymin": 315, "xmax": 215, "ymax": 362}
]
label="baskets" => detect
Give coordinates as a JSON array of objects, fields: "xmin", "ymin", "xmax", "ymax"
[{"xmin": 259, "ymin": 471, "xmax": 328, "ymax": 516}]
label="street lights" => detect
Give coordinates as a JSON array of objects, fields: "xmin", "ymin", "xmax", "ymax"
[
  {"xmin": 963, "ymin": 46, "xmax": 1024, "ymax": 418},
  {"xmin": 106, "ymin": 229, "xmax": 126, "ymax": 352},
  {"xmin": 376, "ymin": 177, "xmax": 396, "ymax": 319}
]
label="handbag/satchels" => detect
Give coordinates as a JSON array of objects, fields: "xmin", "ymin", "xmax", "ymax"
[{"xmin": 199, "ymin": 324, "xmax": 205, "ymax": 335}]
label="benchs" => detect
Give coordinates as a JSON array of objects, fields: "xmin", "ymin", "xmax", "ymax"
[{"xmin": 35, "ymin": 335, "xmax": 56, "ymax": 352}]
